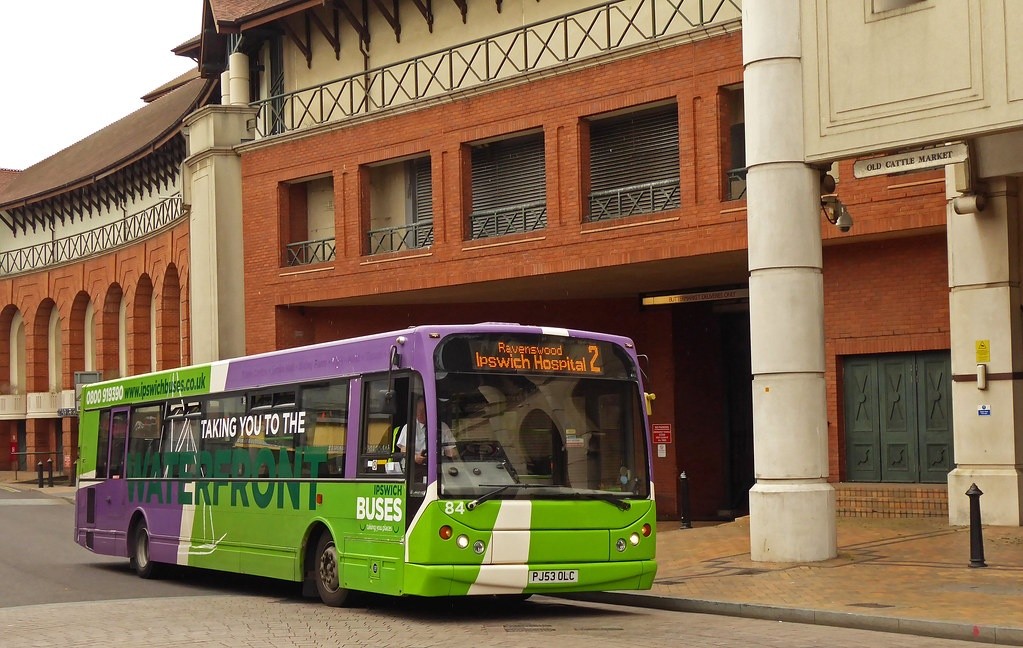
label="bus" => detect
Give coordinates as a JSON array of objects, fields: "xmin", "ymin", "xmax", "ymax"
[{"xmin": 73, "ymin": 323, "xmax": 657, "ymax": 610}]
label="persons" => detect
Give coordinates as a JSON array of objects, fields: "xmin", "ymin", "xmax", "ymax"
[{"xmin": 396, "ymin": 398, "xmax": 459, "ymax": 480}]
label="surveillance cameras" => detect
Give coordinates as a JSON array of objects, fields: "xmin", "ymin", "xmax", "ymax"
[{"xmin": 835, "ymin": 214, "xmax": 853, "ymax": 233}]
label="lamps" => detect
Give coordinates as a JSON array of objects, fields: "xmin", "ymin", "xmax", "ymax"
[{"xmin": 976, "ymin": 364, "xmax": 986, "ymax": 391}]
